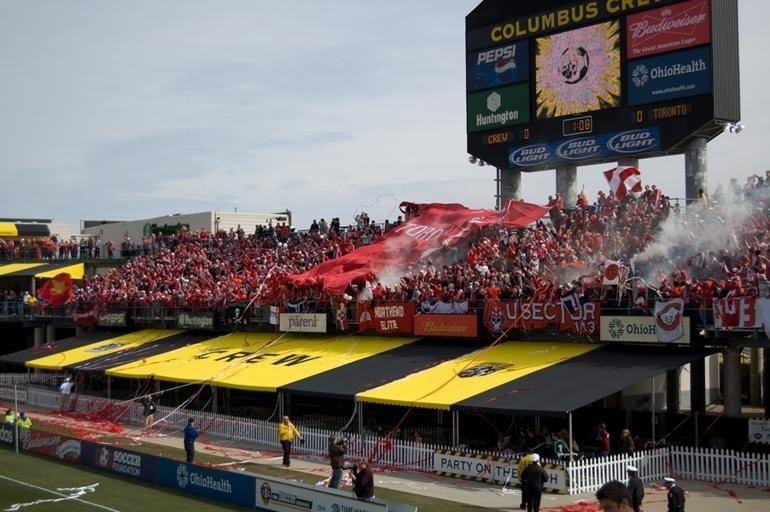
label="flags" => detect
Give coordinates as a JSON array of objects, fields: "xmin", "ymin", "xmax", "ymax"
[{"xmin": 603, "ymin": 166, "xmax": 643, "ymax": 200}]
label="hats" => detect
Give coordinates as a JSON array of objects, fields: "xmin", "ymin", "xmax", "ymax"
[
  {"xmin": 664, "ymin": 478, "xmax": 675, "ymax": 484},
  {"xmin": 625, "ymin": 466, "xmax": 638, "ymax": 472},
  {"xmin": 531, "ymin": 453, "xmax": 540, "ymax": 462}
]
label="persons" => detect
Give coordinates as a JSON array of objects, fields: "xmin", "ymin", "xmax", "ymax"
[
  {"xmin": 143, "ymin": 395, "xmax": 157, "ymax": 434},
  {"xmin": 517, "ymin": 448, "xmax": 536, "ymax": 508},
  {"xmin": 625, "ymin": 465, "xmax": 645, "ymax": 511},
  {"xmin": 16, "ymin": 410, "xmax": 32, "ymax": 434},
  {"xmin": 58, "ymin": 377, "xmax": 77, "ymax": 414},
  {"xmin": 184, "ymin": 418, "xmax": 200, "ymax": 463},
  {"xmin": 349, "ymin": 460, "xmax": 376, "ymax": 501},
  {"xmin": 519, "ymin": 453, "xmax": 549, "ymax": 511},
  {"xmin": 326, "ymin": 433, "xmax": 346, "ymax": 489},
  {"xmin": 664, "ymin": 475, "xmax": 687, "ymax": 511},
  {"xmin": 347, "ymin": 408, "xmax": 637, "ymax": 459},
  {"xmin": 596, "ymin": 478, "xmax": 634, "ymax": 511},
  {"xmin": 5, "ymin": 408, "xmax": 15, "ymax": 430},
  {"xmin": 277, "ymin": 415, "xmax": 305, "ymax": 467},
  {"xmin": 0, "ymin": 171, "xmax": 769, "ymax": 342}
]
